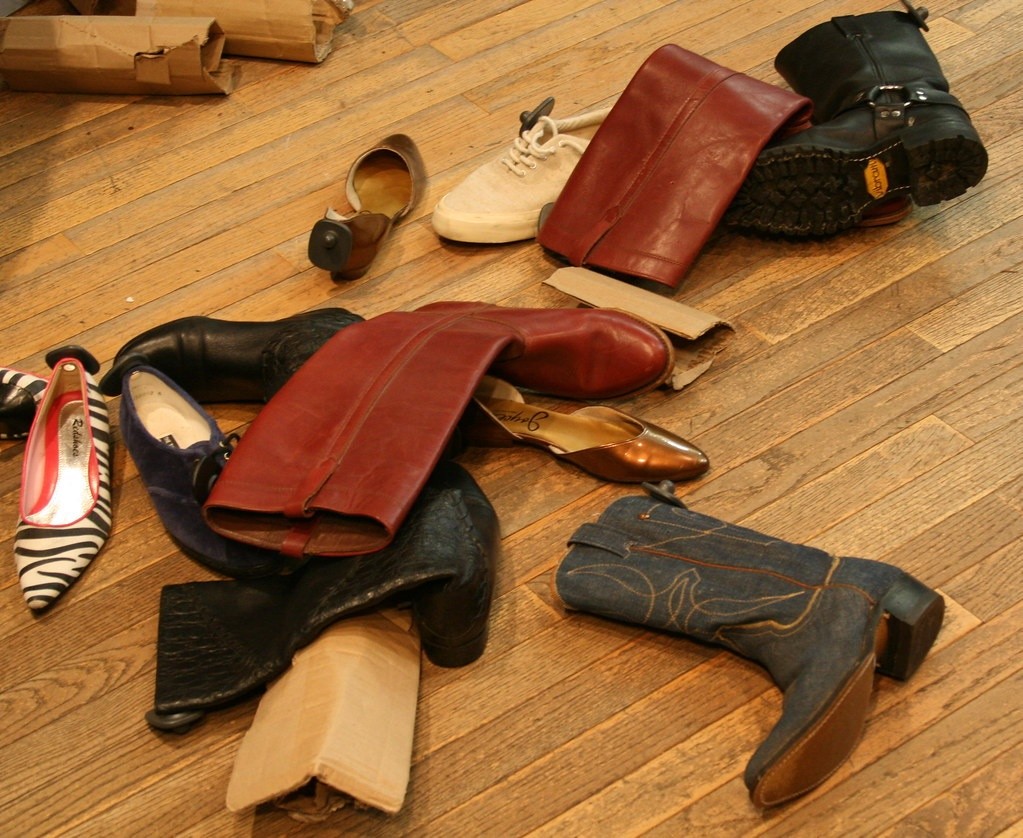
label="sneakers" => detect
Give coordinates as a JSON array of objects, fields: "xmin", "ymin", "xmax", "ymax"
[{"xmin": 432, "ymin": 108, "xmax": 613, "ymax": 243}]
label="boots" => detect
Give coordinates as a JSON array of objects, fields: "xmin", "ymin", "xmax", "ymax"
[
  {"xmin": 154, "ymin": 458, "xmax": 498, "ymax": 715},
  {"xmin": 203, "ymin": 301, "xmax": 675, "ymax": 558},
  {"xmin": 537, "ymin": 43, "xmax": 913, "ymax": 288},
  {"xmin": 719, "ymin": 0, "xmax": 989, "ymax": 235},
  {"xmin": 112, "ymin": 307, "xmax": 365, "ymax": 404},
  {"xmin": 557, "ymin": 496, "xmax": 946, "ymax": 809}
]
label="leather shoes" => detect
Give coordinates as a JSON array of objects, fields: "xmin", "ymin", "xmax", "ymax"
[
  {"xmin": 324, "ymin": 134, "xmax": 427, "ymax": 279},
  {"xmin": 460, "ymin": 375, "xmax": 708, "ymax": 483}
]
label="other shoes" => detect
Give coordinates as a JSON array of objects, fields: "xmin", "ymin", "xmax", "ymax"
[
  {"xmin": 120, "ymin": 362, "xmax": 254, "ymax": 576},
  {"xmin": 0, "ymin": 366, "xmax": 49, "ymax": 439},
  {"xmin": 13, "ymin": 356, "xmax": 111, "ymax": 607}
]
